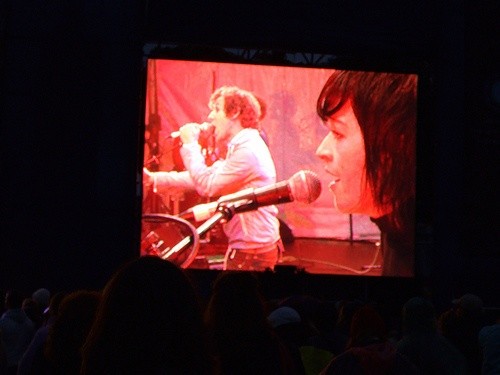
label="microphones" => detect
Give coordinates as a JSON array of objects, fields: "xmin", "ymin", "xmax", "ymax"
[
  {"xmin": 171, "ymin": 122, "xmax": 212, "ymax": 138},
  {"xmin": 176, "ymin": 170, "xmax": 322, "ymax": 225}
]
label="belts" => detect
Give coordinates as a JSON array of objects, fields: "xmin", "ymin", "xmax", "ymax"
[{"xmin": 237, "ymin": 244, "xmax": 278, "ymax": 253}]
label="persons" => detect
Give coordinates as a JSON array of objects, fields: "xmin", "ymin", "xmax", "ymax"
[
  {"xmin": 0, "ymin": 256, "xmax": 500, "ymax": 375},
  {"xmin": 144, "ymin": 71, "xmax": 417, "ymax": 272}
]
